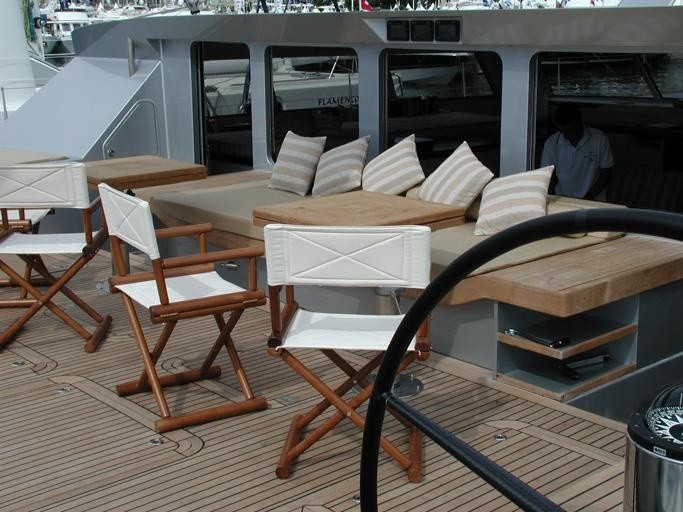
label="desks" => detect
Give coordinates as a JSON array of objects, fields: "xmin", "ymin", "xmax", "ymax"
[{"xmin": 123, "ymin": 171, "xmax": 683, "ymax": 405}]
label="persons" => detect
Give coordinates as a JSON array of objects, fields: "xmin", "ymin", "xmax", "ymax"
[{"xmin": 539, "ymin": 101, "xmax": 618, "ymax": 203}]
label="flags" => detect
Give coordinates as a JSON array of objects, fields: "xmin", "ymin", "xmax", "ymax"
[{"xmin": 360, "ymin": 1, "xmax": 373, "ymax": 12}]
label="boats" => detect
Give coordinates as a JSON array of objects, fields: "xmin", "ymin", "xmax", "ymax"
[{"xmin": 40, "ymin": 8, "xmax": 92, "ymax": 56}]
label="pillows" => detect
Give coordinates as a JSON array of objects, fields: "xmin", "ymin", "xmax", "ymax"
[{"xmin": 267, "ymin": 129, "xmax": 559, "ymax": 234}]
